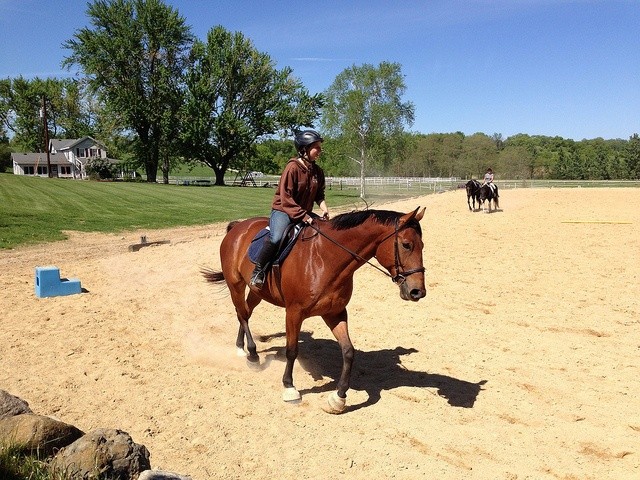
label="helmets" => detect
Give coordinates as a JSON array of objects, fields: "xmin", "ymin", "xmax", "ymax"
[{"xmin": 295, "ymin": 131, "xmax": 324, "ymax": 152}]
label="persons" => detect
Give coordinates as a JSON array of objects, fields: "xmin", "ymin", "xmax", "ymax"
[
  {"xmin": 249, "ymin": 130, "xmax": 330, "ymax": 290},
  {"xmin": 484, "ymin": 168, "xmax": 496, "ymax": 190}
]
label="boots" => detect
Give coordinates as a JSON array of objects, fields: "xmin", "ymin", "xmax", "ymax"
[{"xmin": 250, "ymin": 240, "xmax": 277, "ymax": 283}]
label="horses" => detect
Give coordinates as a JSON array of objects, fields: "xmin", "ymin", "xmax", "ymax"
[
  {"xmin": 464, "ymin": 178, "xmax": 482, "ymax": 210},
  {"xmin": 198, "ymin": 205, "xmax": 426, "ymax": 415},
  {"xmin": 478, "ymin": 182, "xmax": 499, "ymax": 213}
]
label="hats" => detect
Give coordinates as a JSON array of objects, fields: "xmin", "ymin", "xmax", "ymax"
[{"xmin": 487, "ymin": 168, "xmax": 492, "ymax": 171}]
